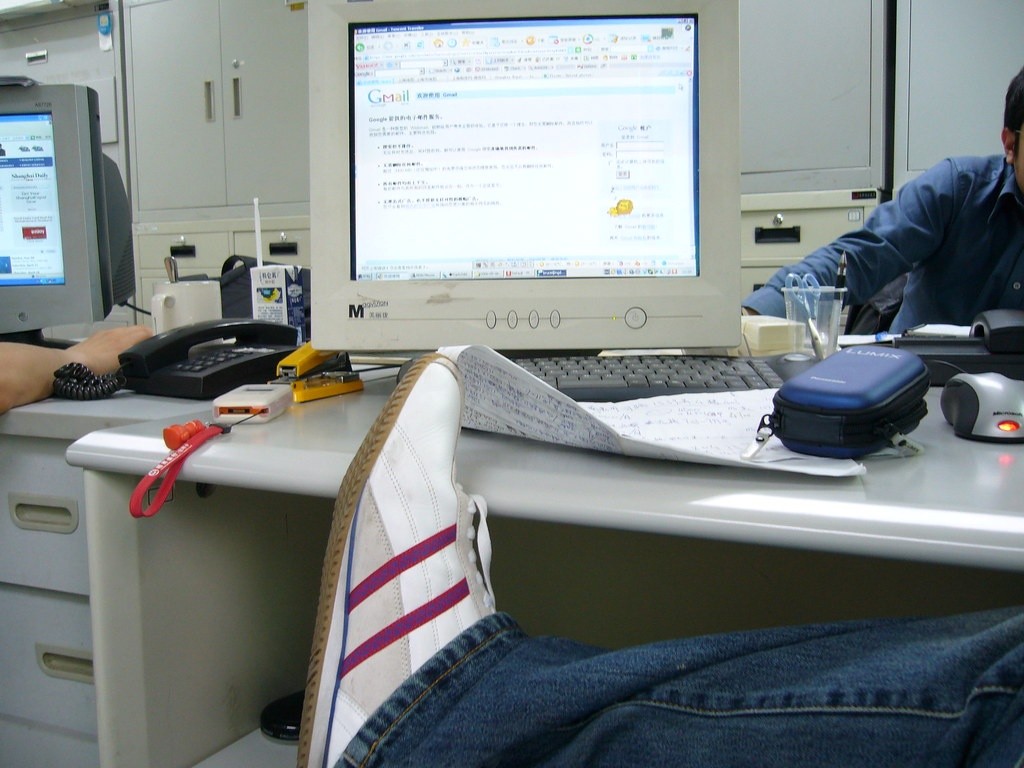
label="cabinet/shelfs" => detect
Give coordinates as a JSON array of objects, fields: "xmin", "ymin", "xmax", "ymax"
[
  {"xmin": 131, "ymin": 215, "xmax": 310, "ymax": 332},
  {"xmin": 0, "ymin": 388, "xmax": 215, "ymax": 768},
  {"xmin": 892, "ymin": 0, "xmax": 1024, "ymax": 198},
  {"xmin": 745, "ymin": 188, "xmax": 885, "ymax": 301},
  {"xmin": 739, "ymin": 0, "xmax": 896, "ymax": 195},
  {"xmin": 122, "ymin": 0, "xmax": 311, "ymax": 220}
]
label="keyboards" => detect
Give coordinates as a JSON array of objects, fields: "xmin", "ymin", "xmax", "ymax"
[{"xmin": 511, "ymin": 352, "xmax": 819, "ymax": 401}]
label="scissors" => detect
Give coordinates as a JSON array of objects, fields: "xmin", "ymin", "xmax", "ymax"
[{"xmin": 783, "ymin": 272, "xmax": 825, "ymax": 360}]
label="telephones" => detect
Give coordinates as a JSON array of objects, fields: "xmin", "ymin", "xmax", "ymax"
[{"xmin": 116, "ymin": 317, "xmax": 303, "ymax": 402}]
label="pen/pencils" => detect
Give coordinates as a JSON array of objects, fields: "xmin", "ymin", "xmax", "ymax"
[
  {"xmin": 875, "ymin": 330, "xmax": 889, "ymax": 342},
  {"xmin": 826, "ymin": 249, "xmax": 849, "ymax": 357}
]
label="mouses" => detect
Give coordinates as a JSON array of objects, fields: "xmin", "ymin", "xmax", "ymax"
[{"xmin": 940, "ymin": 371, "xmax": 1024, "ymax": 444}]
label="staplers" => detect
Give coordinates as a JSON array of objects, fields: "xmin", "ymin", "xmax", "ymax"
[{"xmin": 276, "ymin": 339, "xmax": 366, "ymax": 403}]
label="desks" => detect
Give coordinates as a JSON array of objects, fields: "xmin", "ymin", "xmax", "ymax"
[{"xmin": 76, "ymin": 375, "xmax": 1024, "ymax": 768}]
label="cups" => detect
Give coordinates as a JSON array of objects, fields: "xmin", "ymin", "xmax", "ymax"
[
  {"xmin": 782, "ymin": 285, "xmax": 848, "ymax": 358},
  {"xmin": 152, "ymin": 281, "xmax": 221, "ymax": 336}
]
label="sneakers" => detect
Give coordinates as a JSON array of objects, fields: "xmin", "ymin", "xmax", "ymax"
[{"xmin": 294, "ymin": 350, "xmax": 498, "ymax": 768}]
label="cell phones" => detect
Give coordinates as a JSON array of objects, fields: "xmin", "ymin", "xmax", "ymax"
[{"xmin": 212, "ymin": 384, "xmax": 294, "ymax": 424}]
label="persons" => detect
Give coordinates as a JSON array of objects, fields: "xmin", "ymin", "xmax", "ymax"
[
  {"xmin": 0, "ymin": 325, "xmax": 153, "ymax": 416},
  {"xmin": 741, "ymin": 66, "xmax": 1024, "ymax": 334},
  {"xmin": 296, "ymin": 353, "xmax": 1024, "ymax": 768}
]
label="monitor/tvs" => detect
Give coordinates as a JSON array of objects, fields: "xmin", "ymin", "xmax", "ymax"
[
  {"xmin": 0, "ymin": 85, "xmax": 137, "ymax": 348},
  {"xmin": 308, "ymin": 0, "xmax": 739, "ymax": 361}
]
